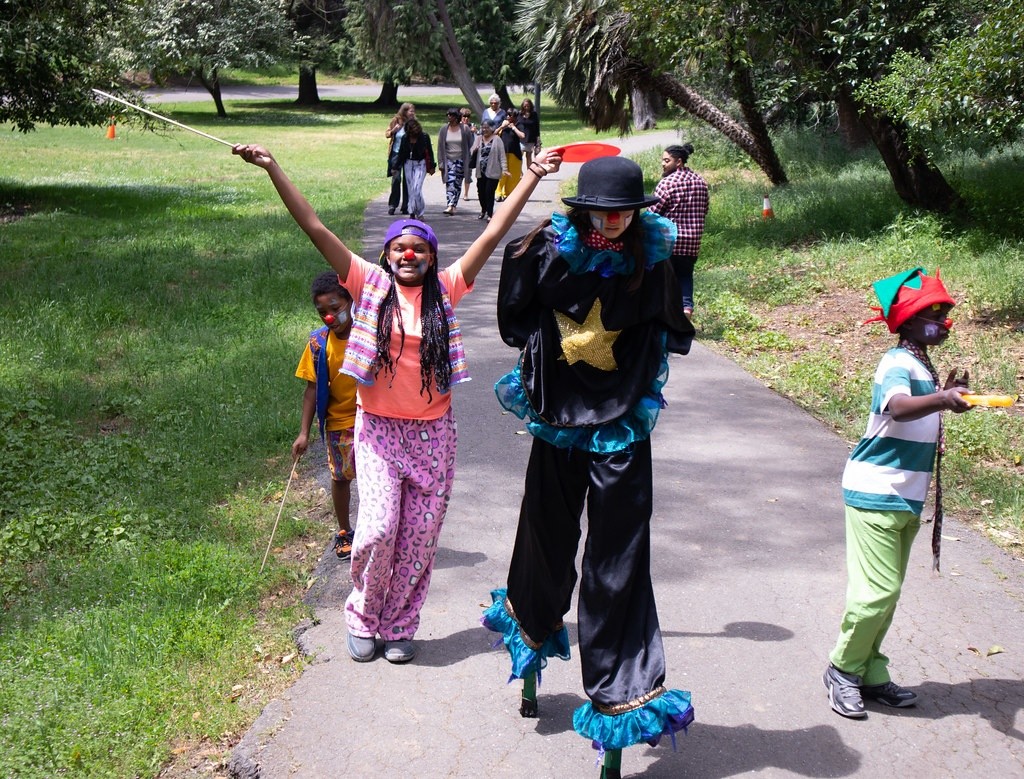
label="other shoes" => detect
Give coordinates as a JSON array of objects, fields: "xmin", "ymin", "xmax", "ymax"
[
  {"xmin": 478, "ymin": 212, "xmax": 491, "ymax": 223},
  {"xmin": 383, "ymin": 638, "xmax": 416, "ymax": 663},
  {"xmin": 443, "ymin": 206, "xmax": 454, "ymax": 215},
  {"xmin": 410, "ymin": 215, "xmax": 424, "ymax": 221},
  {"xmin": 683, "ymin": 306, "xmax": 693, "ymax": 321},
  {"xmin": 388, "ymin": 209, "xmax": 395, "ymax": 215},
  {"xmin": 402, "ymin": 209, "xmax": 409, "ymax": 215},
  {"xmin": 345, "ymin": 630, "xmax": 377, "ymax": 662}
]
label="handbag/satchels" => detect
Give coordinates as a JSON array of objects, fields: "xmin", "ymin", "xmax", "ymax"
[{"xmin": 468, "ymin": 148, "xmax": 478, "ymax": 168}]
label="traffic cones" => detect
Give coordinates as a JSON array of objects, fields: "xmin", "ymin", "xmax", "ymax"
[
  {"xmin": 763, "ymin": 193, "xmax": 775, "ymax": 223},
  {"xmin": 104, "ymin": 115, "xmax": 115, "ymax": 140}
]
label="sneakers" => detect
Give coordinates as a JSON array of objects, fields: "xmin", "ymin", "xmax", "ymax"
[
  {"xmin": 821, "ymin": 661, "xmax": 868, "ymax": 718},
  {"xmin": 862, "ymin": 682, "xmax": 917, "ymax": 709},
  {"xmin": 331, "ymin": 528, "xmax": 355, "ymax": 560}
]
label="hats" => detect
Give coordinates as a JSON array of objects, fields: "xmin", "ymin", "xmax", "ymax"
[
  {"xmin": 863, "ymin": 266, "xmax": 956, "ymax": 334},
  {"xmin": 561, "ymin": 155, "xmax": 663, "ymax": 210},
  {"xmin": 378, "ymin": 219, "xmax": 438, "ymax": 267}
]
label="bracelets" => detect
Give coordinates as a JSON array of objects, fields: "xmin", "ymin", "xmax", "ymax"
[
  {"xmin": 512, "ymin": 126, "xmax": 516, "ymax": 130},
  {"xmin": 528, "ymin": 167, "xmax": 542, "ymax": 179},
  {"xmin": 532, "ymin": 162, "xmax": 548, "ymax": 176}
]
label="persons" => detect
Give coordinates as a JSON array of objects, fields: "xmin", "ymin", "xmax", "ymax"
[
  {"xmin": 385, "ymin": 103, "xmax": 418, "ymax": 215},
  {"xmin": 479, "ymin": 156, "xmax": 696, "ymax": 747},
  {"xmin": 822, "ymin": 266, "xmax": 979, "ymax": 718},
  {"xmin": 231, "ymin": 142, "xmax": 565, "ymax": 663},
  {"xmin": 647, "ymin": 144, "xmax": 709, "ymax": 321},
  {"xmin": 293, "ymin": 272, "xmax": 358, "ymax": 560},
  {"xmin": 438, "ymin": 108, "xmax": 474, "ymax": 216},
  {"xmin": 460, "ymin": 94, "xmax": 543, "ymax": 223},
  {"xmin": 392, "ymin": 119, "xmax": 435, "ymax": 222}
]
